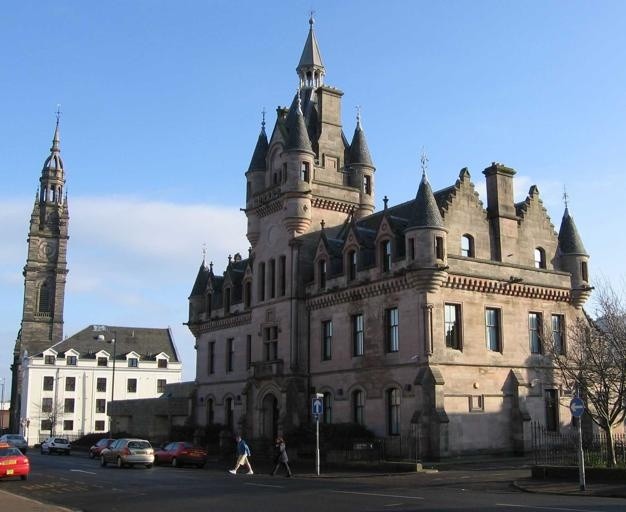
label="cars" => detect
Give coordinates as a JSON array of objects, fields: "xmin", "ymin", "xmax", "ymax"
[
  {"xmin": 314, "ymin": 437, "xmax": 384, "ymax": 469},
  {"xmin": 154, "ymin": 442, "xmax": 208, "ymax": 468},
  {"xmin": 0, "ymin": 442, "xmax": 30, "ymax": 481},
  {"xmin": 0, "ymin": 434, "xmax": 28, "ymax": 454},
  {"xmin": 41, "ymin": 438, "xmax": 71, "ymax": 456},
  {"xmin": 89, "ymin": 439, "xmax": 117, "ymax": 459},
  {"xmin": 99, "ymin": 439, "xmax": 155, "ymax": 469}
]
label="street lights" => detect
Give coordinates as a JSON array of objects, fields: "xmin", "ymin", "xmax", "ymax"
[
  {"xmin": 0, "ymin": 377, "xmax": 4, "ymax": 433},
  {"xmin": 109, "ymin": 337, "xmax": 117, "ymax": 438}
]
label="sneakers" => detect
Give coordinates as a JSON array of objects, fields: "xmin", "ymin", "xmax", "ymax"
[
  {"xmin": 270, "ymin": 472, "xmax": 276, "ymax": 476},
  {"xmin": 285, "ymin": 475, "xmax": 292, "ymax": 478},
  {"xmin": 247, "ymin": 471, "xmax": 253, "ymax": 474},
  {"xmin": 229, "ymin": 470, "xmax": 236, "ymax": 475}
]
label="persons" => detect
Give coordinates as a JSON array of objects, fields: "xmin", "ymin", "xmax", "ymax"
[
  {"xmin": 227, "ymin": 433, "xmax": 254, "ymax": 474},
  {"xmin": 270, "ymin": 436, "xmax": 293, "ymax": 478}
]
